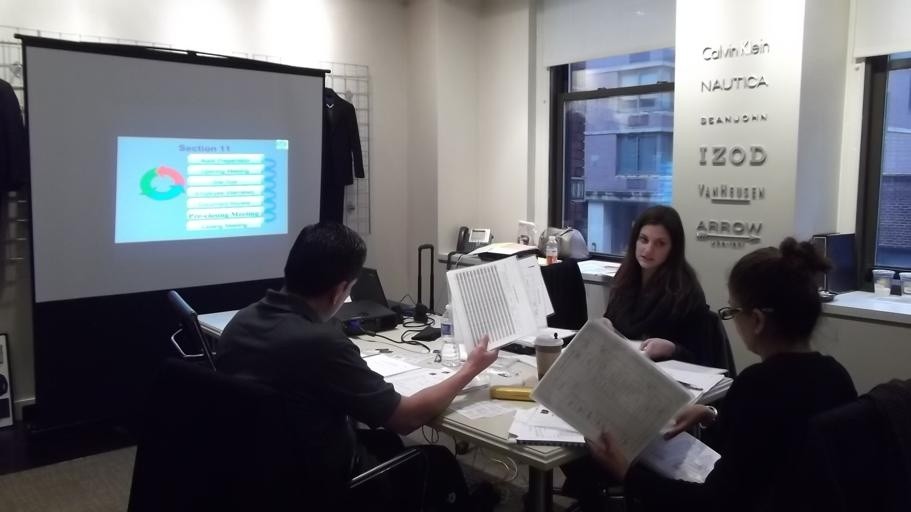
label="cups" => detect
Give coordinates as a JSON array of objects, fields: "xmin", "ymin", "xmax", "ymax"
[
  {"xmin": 898, "ymin": 271, "xmax": 911, "ymax": 303},
  {"xmin": 871, "ymin": 268, "xmax": 896, "ymax": 299},
  {"xmin": 533, "ymin": 335, "xmax": 565, "ymax": 381}
]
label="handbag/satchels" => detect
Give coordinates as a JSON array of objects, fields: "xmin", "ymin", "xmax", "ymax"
[{"xmin": 537, "ymin": 226, "xmax": 588, "ymax": 260}]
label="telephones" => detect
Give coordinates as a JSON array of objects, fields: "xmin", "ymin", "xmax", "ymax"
[{"xmin": 457, "ymin": 226, "xmax": 494, "ymax": 254}]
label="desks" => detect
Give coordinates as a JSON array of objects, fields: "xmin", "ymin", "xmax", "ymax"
[{"xmin": 814, "ymin": 291, "xmax": 911, "ymax": 395}]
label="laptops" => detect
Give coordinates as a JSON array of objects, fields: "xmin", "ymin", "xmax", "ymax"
[{"xmin": 349, "ymin": 266, "xmax": 411, "ymax": 309}]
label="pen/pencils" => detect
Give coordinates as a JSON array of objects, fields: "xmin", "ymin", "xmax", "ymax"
[{"xmin": 679, "ymin": 380, "xmax": 703, "ymax": 390}]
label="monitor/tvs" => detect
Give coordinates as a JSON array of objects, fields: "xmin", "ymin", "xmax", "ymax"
[{"xmin": 516, "ymin": 221, "xmax": 536, "ymax": 245}]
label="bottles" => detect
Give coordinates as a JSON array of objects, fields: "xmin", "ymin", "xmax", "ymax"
[
  {"xmin": 441, "ymin": 305, "xmax": 461, "ymax": 368},
  {"xmin": 545, "ymin": 235, "xmax": 558, "ymax": 265}
]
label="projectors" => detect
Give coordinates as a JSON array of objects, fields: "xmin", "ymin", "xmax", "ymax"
[{"xmin": 333, "ymin": 300, "xmax": 399, "ymax": 337}]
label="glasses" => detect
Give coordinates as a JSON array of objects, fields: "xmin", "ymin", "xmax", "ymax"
[{"xmin": 717, "ymin": 305, "xmax": 773, "ymax": 321}]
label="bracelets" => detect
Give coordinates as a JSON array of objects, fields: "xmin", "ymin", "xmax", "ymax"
[{"xmin": 698, "ymin": 406, "xmax": 720, "ymax": 432}]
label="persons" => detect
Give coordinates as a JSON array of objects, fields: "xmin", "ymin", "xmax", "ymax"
[
  {"xmin": 601, "ymin": 204, "xmax": 724, "ymax": 369},
  {"xmin": 584, "ymin": 236, "xmax": 857, "ymax": 512},
  {"xmin": 212, "ymin": 218, "xmax": 499, "ymax": 512}
]
